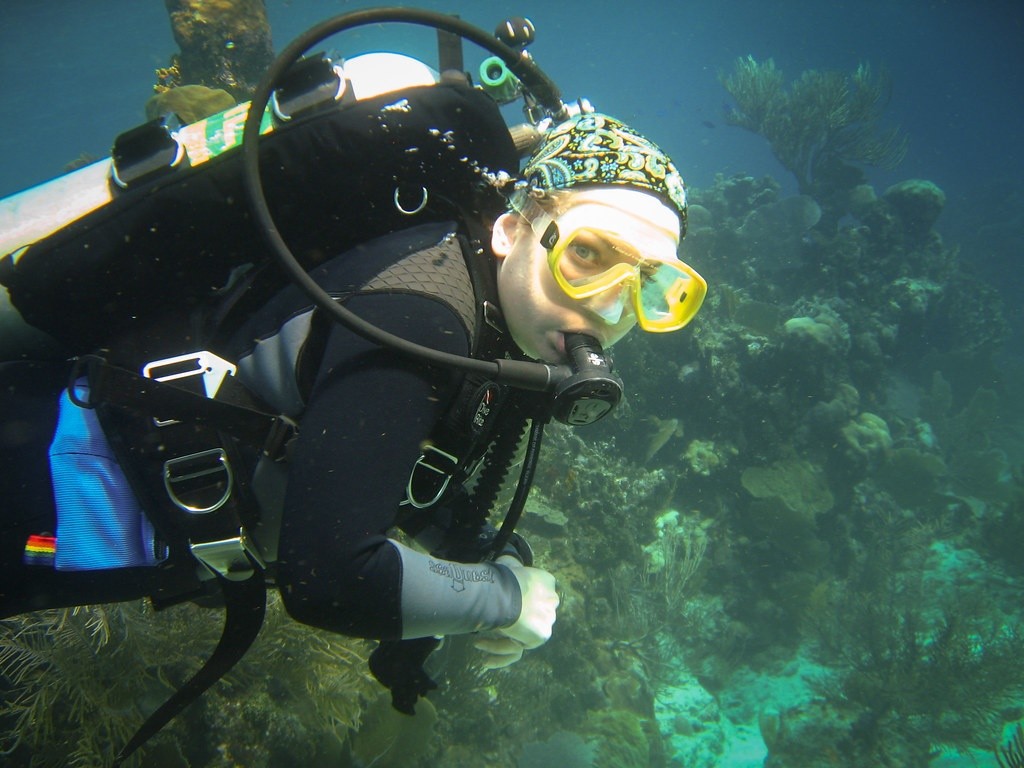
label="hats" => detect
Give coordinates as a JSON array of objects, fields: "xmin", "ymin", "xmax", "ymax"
[{"xmin": 513, "ymin": 112, "xmax": 689, "ymax": 242}]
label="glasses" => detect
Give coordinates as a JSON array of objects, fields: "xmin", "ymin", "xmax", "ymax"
[{"xmin": 549, "ymin": 225, "xmax": 708, "ymax": 335}]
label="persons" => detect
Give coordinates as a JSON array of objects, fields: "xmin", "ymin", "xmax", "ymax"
[{"xmin": 1, "ymin": 114, "xmax": 688, "ymax": 669}]
